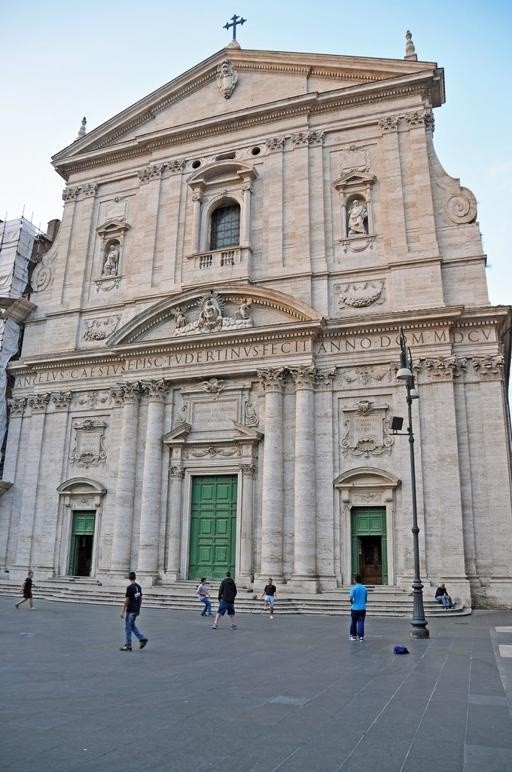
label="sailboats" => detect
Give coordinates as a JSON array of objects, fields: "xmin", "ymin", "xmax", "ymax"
[{"xmin": 252, "ymin": 595, "xmax": 257, "ymax": 600}]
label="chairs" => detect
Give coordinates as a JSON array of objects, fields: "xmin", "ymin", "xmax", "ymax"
[
  {"xmin": 230, "ymin": 624, "xmax": 237, "ymax": 627},
  {"xmin": 210, "ymin": 624, "xmax": 218, "ymax": 629},
  {"xmin": 349, "ymin": 636, "xmax": 364, "ymax": 641}
]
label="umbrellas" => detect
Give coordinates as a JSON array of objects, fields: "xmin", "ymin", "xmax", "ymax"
[
  {"xmin": 120, "ymin": 644, "xmax": 132, "ymax": 651},
  {"xmin": 139, "ymin": 639, "xmax": 148, "ymax": 650}
]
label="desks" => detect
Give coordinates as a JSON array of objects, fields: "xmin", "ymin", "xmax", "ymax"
[{"xmin": 395, "ymin": 327, "xmax": 429, "ymax": 638}]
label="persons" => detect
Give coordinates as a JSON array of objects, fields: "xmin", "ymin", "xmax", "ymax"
[
  {"xmin": 195, "ymin": 577, "xmax": 213, "ymax": 617},
  {"xmin": 260, "ymin": 577, "xmax": 278, "ymax": 619},
  {"xmin": 204, "ymin": 300, "xmax": 217, "ymax": 323},
  {"xmin": 434, "ymin": 583, "xmax": 453, "ymax": 612},
  {"xmin": 209, "ymin": 571, "xmax": 237, "ymax": 630},
  {"xmin": 103, "ymin": 244, "xmax": 119, "ymax": 276},
  {"xmin": 234, "ymin": 298, "xmax": 251, "ymax": 320},
  {"xmin": 348, "ymin": 199, "xmax": 369, "ymax": 236},
  {"xmin": 346, "ymin": 574, "xmax": 368, "ymax": 641},
  {"xmin": 175, "ymin": 307, "xmax": 188, "ymax": 328},
  {"xmin": 120, "ymin": 572, "xmax": 148, "ymax": 651},
  {"xmin": 14, "ymin": 571, "xmax": 38, "ymax": 611}
]
